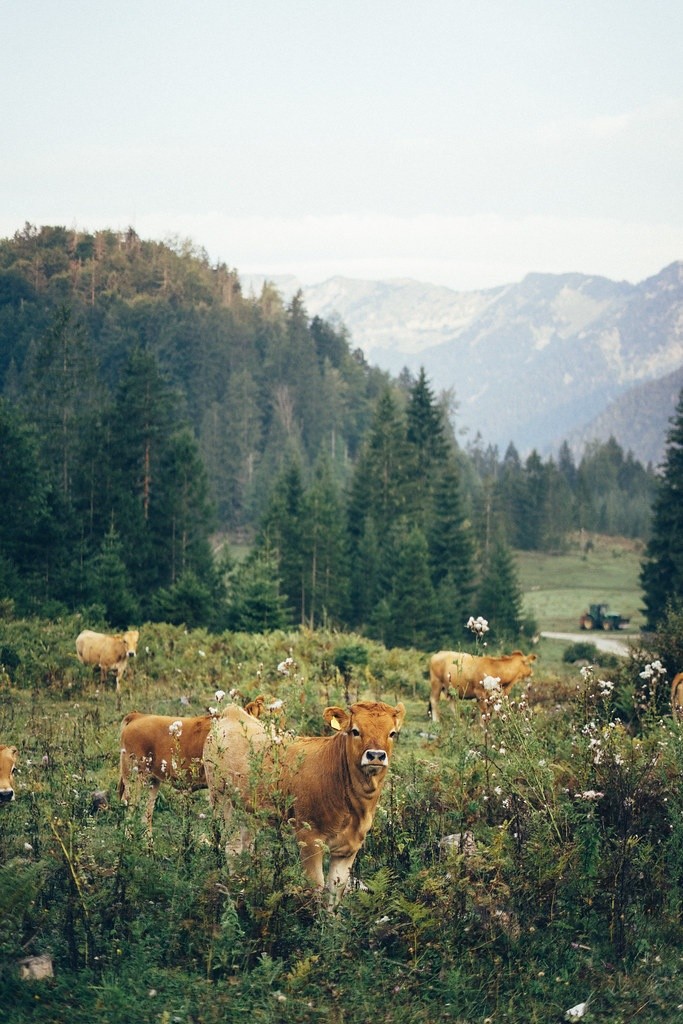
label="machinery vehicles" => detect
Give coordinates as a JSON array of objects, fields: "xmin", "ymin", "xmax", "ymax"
[{"xmin": 581, "ymin": 604, "xmax": 630, "ymax": 631}]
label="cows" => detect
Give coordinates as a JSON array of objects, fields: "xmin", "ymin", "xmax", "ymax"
[
  {"xmin": 116, "ymin": 695, "xmax": 287, "ymax": 850},
  {"xmin": 0, "ymin": 744, "xmax": 19, "ymax": 803},
  {"xmin": 202, "ymin": 699, "xmax": 407, "ymax": 914},
  {"xmin": 76, "ymin": 630, "xmax": 140, "ymax": 694},
  {"xmin": 428, "ymin": 650, "xmax": 537, "ymax": 723}
]
